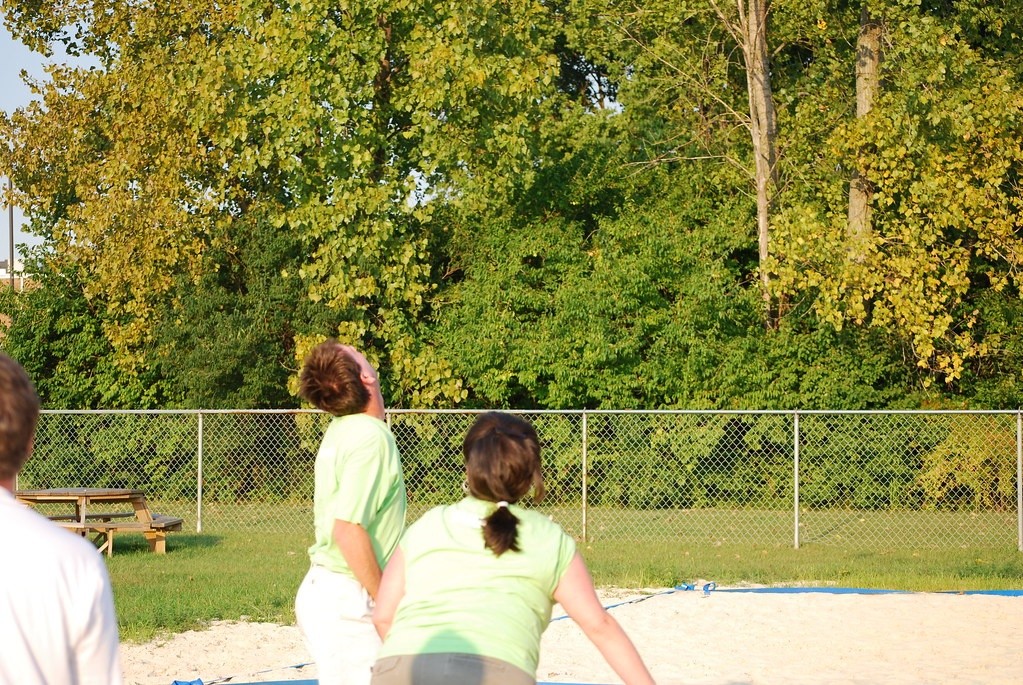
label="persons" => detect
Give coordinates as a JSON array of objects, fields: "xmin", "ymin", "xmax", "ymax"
[
  {"xmin": 371, "ymin": 412, "xmax": 657, "ymax": 685},
  {"xmin": 294, "ymin": 342, "xmax": 408, "ymax": 685},
  {"xmin": 0, "ymin": 354, "xmax": 123, "ymax": 685}
]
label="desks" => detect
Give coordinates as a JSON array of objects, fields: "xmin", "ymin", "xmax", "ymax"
[{"xmin": 17, "ymin": 487, "xmax": 157, "ymax": 553}]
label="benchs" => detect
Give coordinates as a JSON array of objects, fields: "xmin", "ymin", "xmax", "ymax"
[{"xmin": 50, "ymin": 513, "xmax": 185, "ymax": 559}]
